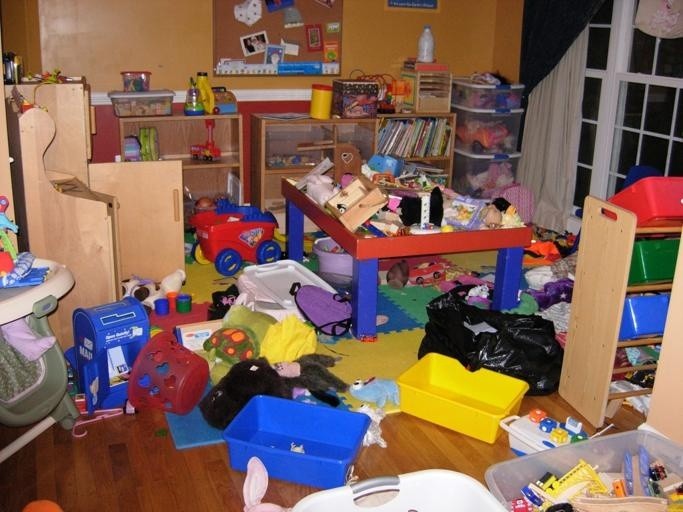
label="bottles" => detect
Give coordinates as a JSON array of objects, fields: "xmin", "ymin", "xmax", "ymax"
[
  {"xmin": 157, "ymin": 269, "xmax": 186, "ymax": 292},
  {"xmin": 417, "ymin": 25, "xmax": 434, "ymax": 63},
  {"xmin": 310, "ymin": 84, "xmax": 333, "ymax": 120}
]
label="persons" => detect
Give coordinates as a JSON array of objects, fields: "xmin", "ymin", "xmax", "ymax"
[{"xmin": 242, "ymin": 34, "xmax": 267, "ymax": 54}]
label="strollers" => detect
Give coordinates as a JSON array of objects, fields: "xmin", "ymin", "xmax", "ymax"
[{"xmin": 0, "ymin": 196, "xmax": 78, "ymax": 463}]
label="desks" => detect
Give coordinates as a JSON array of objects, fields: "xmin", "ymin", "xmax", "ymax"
[{"xmin": 277, "ymin": 175, "xmax": 534, "ymax": 338}]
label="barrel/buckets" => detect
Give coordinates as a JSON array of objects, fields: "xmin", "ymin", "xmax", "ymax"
[{"xmin": 196, "ymin": 69, "xmax": 214, "ymax": 115}]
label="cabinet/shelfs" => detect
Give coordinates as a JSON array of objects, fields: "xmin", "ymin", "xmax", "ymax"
[
  {"xmin": 249, "ymin": 113, "xmax": 376, "ymax": 214},
  {"xmin": 118, "ymin": 116, "xmax": 244, "ymax": 222},
  {"xmin": 376, "ymin": 116, "xmax": 455, "ymax": 188},
  {"xmin": 559, "ymin": 195, "xmax": 683, "ymax": 426},
  {"xmin": 6, "ymin": 77, "xmax": 185, "ymax": 354}
]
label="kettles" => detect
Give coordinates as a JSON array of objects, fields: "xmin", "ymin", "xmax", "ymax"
[{"xmin": 4, "ymin": 50, "xmax": 21, "ymax": 84}]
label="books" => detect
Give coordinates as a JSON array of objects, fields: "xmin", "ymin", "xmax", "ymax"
[
  {"xmin": 377, "ymin": 118, "xmax": 452, "ymax": 157},
  {"xmin": 407, "ymin": 162, "xmax": 444, "ymax": 175}
]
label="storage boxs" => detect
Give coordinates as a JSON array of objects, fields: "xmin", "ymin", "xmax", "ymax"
[
  {"xmin": 292, "ymin": 468, "xmax": 510, "ymax": 512},
  {"xmin": 486, "ymin": 428, "xmax": 683, "ymax": 512},
  {"xmin": 602, "ymin": 174, "xmax": 682, "ymax": 339},
  {"xmin": 498, "ymin": 413, "xmax": 592, "ymax": 458},
  {"xmin": 452, "ymin": 77, "xmax": 525, "ymax": 201},
  {"xmin": 396, "ymin": 352, "xmax": 530, "ymax": 446},
  {"xmin": 223, "ymin": 395, "xmax": 371, "ymax": 489},
  {"xmin": 109, "ymin": 90, "xmax": 175, "ymax": 116}
]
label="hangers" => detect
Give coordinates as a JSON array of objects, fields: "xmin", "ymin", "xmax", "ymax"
[{"xmin": 68, "ymin": 407, "xmax": 123, "ymax": 438}]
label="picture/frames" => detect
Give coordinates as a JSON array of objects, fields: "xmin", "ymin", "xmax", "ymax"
[{"xmin": 384, "ymin": 0, "xmax": 441, "ymax": 13}]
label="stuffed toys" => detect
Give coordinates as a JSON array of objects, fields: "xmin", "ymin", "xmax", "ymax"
[
  {"xmin": 0, "ymin": 195, "xmax": 50, "ymax": 286},
  {"xmin": 243, "ymin": 456, "xmax": 289, "ymax": 512},
  {"xmin": 198, "ymin": 354, "xmax": 400, "ymax": 448}
]
